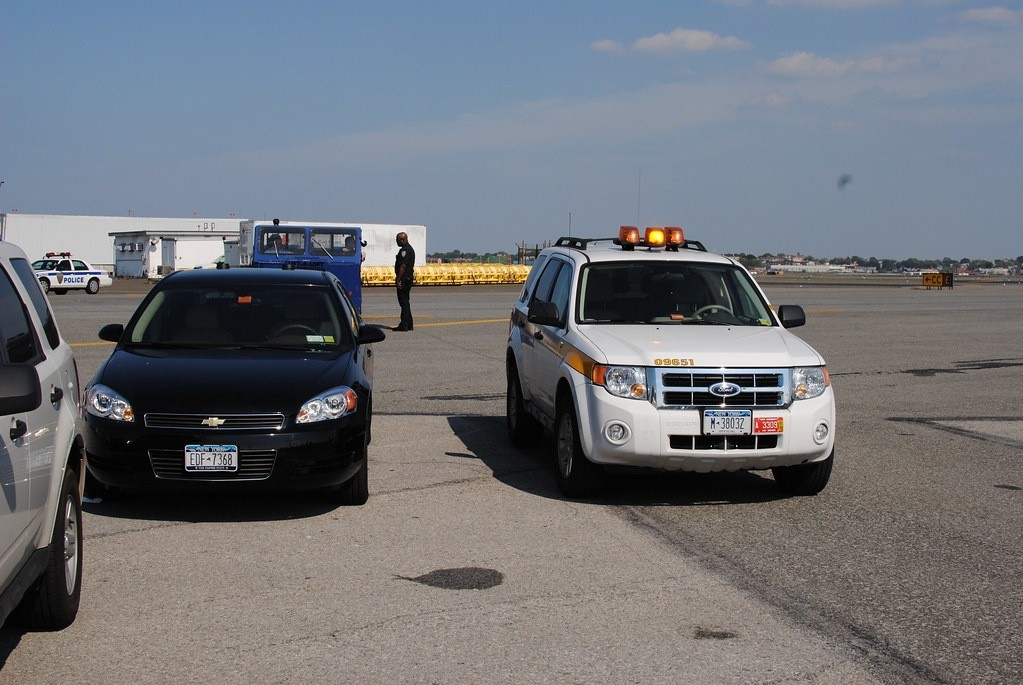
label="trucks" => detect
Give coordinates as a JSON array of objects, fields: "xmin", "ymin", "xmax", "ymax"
[{"xmin": 240, "ymin": 217, "xmax": 367, "ymax": 324}]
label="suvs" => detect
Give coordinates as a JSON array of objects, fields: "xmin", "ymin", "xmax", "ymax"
[
  {"xmin": 506, "ymin": 225, "xmax": 837, "ymax": 501},
  {"xmin": 0, "ymin": 241, "xmax": 87, "ymax": 635}
]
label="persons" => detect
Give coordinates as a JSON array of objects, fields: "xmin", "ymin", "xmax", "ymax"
[
  {"xmin": 340, "ymin": 236, "xmax": 356, "ymax": 256},
  {"xmin": 263, "ymin": 234, "xmax": 288, "ymax": 253},
  {"xmin": 392, "ymin": 232, "xmax": 415, "ymax": 331}
]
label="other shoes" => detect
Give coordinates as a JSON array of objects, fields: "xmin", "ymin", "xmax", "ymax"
[
  {"xmin": 408, "ymin": 325, "xmax": 413, "ymax": 330},
  {"xmin": 393, "ymin": 325, "xmax": 408, "ymax": 331}
]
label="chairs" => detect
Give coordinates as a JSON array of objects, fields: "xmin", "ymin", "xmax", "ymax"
[
  {"xmin": 587, "ymin": 277, "xmax": 638, "ymax": 317},
  {"xmin": 660, "ymin": 275, "xmax": 704, "ymax": 316},
  {"xmin": 270, "ymin": 298, "xmax": 333, "ymax": 338},
  {"xmin": 171, "ymin": 307, "xmax": 235, "ymax": 344}
]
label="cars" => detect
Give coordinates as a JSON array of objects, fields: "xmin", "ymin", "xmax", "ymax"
[
  {"xmin": 82, "ymin": 262, "xmax": 387, "ymax": 507},
  {"xmin": 28, "ymin": 251, "xmax": 113, "ymax": 295}
]
